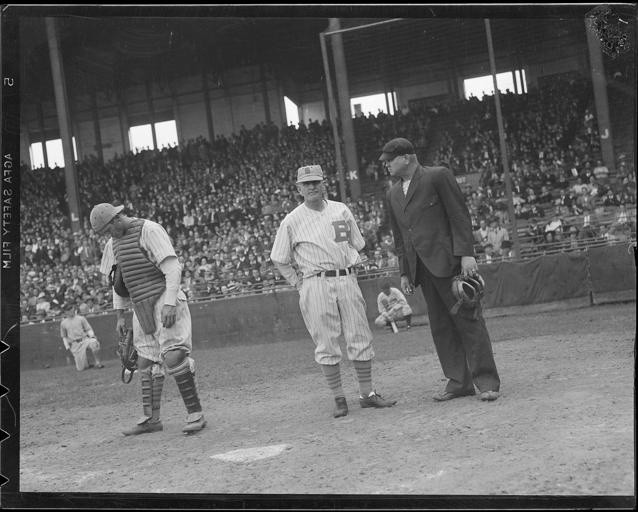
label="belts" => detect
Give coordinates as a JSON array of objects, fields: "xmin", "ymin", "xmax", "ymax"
[{"xmin": 318, "ymin": 268, "xmax": 354, "ymax": 276}]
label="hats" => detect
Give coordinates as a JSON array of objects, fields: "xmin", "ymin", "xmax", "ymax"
[
  {"xmin": 378, "ymin": 138, "xmax": 417, "ymax": 162},
  {"xmin": 90, "ymin": 203, "xmax": 125, "ymax": 232},
  {"xmin": 297, "ymin": 165, "xmax": 324, "ymax": 183}
]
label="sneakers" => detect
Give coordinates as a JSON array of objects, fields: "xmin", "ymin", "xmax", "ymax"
[
  {"xmin": 183, "ymin": 413, "xmax": 206, "ymax": 431},
  {"xmin": 360, "ymin": 390, "xmax": 397, "ymax": 407},
  {"xmin": 333, "ymin": 398, "xmax": 348, "ymax": 418},
  {"xmin": 122, "ymin": 420, "xmax": 162, "ymax": 435},
  {"xmin": 432, "ymin": 389, "xmax": 475, "ymax": 401},
  {"xmin": 479, "ymin": 390, "xmax": 500, "ymax": 400}
]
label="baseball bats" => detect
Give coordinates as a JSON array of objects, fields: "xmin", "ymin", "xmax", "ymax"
[{"xmin": 383, "ymin": 308, "xmax": 399, "ymax": 334}]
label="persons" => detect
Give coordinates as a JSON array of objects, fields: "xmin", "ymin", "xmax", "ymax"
[{"xmin": 21, "ymin": 67, "xmax": 637, "ymax": 437}]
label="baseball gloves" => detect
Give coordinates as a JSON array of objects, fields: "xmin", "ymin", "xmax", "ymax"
[
  {"xmin": 118, "ymin": 327, "xmax": 139, "ymax": 369},
  {"xmin": 452, "ymin": 272, "xmax": 487, "ymax": 307}
]
